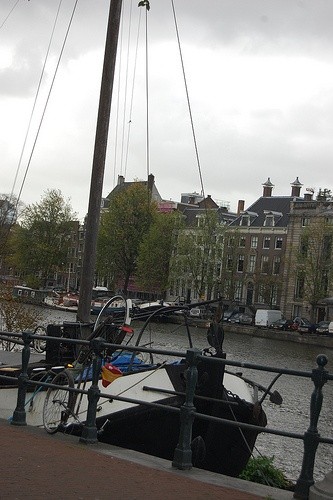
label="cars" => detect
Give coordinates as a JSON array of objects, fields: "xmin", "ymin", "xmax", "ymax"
[
  {"xmin": 222, "ymin": 313, "xmax": 253, "ymax": 326},
  {"xmin": 270, "ymin": 318, "xmax": 333, "ymax": 336},
  {"xmin": 173, "ymin": 308, "xmax": 211, "ymax": 318}
]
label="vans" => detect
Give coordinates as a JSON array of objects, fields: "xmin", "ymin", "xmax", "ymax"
[{"xmin": 254, "ymin": 309, "xmax": 287, "ymax": 329}]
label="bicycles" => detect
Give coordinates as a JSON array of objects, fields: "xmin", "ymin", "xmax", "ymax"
[{"xmin": 43, "ymin": 295, "xmax": 133, "ymax": 434}]
